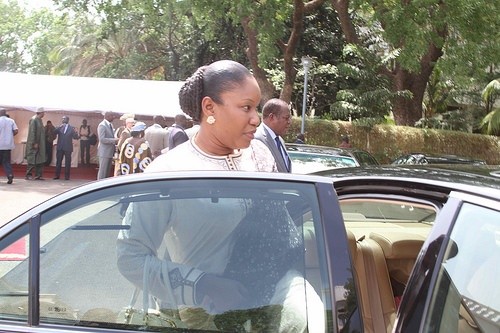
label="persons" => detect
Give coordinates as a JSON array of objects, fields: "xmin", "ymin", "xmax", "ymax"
[
  {"xmin": 0, "ymin": 108, "xmax": 18, "ymax": 183},
  {"xmin": 79, "ymin": 119, "xmax": 93, "ymax": 167},
  {"xmin": 97, "ymin": 111, "xmax": 119, "ymax": 180},
  {"xmin": 114, "ymin": 115, "xmax": 201, "ymax": 215},
  {"xmin": 43, "ymin": 120, "xmax": 57, "ymax": 165},
  {"xmin": 338, "ymin": 138, "xmax": 349, "ymax": 147},
  {"xmin": 114, "ymin": 61, "xmax": 303, "ymax": 333},
  {"xmin": 251, "ymin": 99, "xmax": 292, "ymax": 174},
  {"xmin": 54, "ymin": 116, "xmax": 81, "ymax": 180},
  {"xmin": 24, "ymin": 107, "xmax": 46, "ymax": 180}
]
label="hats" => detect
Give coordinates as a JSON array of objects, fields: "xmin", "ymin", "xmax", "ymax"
[
  {"xmin": 120, "ymin": 113, "xmax": 135, "ymax": 123},
  {"xmin": 33, "ymin": 107, "xmax": 47, "ymax": 113},
  {"xmin": 131, "ymin": 121, "xmax": 146, "ymax": 131}
]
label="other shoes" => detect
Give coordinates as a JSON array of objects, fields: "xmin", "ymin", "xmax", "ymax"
[
  {"xmin": 25, "ymin": 175, "xmax": 33, "ymax": 180},
  {"xmin": 7, "ymin": 176, "xmax": 13, "ymax": 184},
  {"xmin": 35, "ymin": 175, "xmax": 40, "ymax": 180},
  {"xmin": 65, "ymin": 177, "xmax": 69, "ymax": 180},
  {"xmin": 53, "ymin": 177, "xmax": 59, "ymax": 179}
]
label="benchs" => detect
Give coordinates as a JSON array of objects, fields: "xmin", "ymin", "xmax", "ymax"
[{"xmin": 303, "ymin": 229, "xmax": 424, "ymax": 332}]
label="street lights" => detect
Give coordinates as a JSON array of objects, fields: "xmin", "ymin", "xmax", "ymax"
[{"xmin": 296, "ymin": 57, "xmax": 313, "ymax": 143}]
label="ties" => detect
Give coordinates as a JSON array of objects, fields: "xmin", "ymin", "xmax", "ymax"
[
  {"xmin": 275, "ymin": 136, "xmax": 289, "ymax": 173},
  {"xmin": 61, "ymin": 125, "xmax": 66, "ymax": 134}
]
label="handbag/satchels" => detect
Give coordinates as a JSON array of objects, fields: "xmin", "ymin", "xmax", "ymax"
[
  {"xmin": 88, "ymin": 126, "xmax": 96, "ymax": 145},
  {"xmin": 115, "ymin": 255, "xmax": 188, "ymax": 328}
]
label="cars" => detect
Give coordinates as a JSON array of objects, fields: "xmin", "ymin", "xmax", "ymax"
[
  {"xmin": 278, "ymin": 143, "xmax": 380, "ymax": 175},
  {"xmin": 0, "ymin": 161, "xmax": 500, "ymax": 333},
  {"xmin": 389, "ymin": 154, "xmax": 489, "ymax": 171}
]
